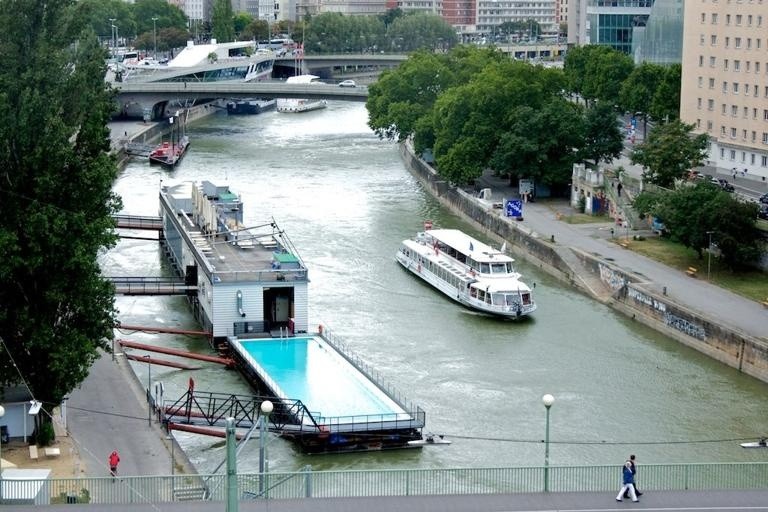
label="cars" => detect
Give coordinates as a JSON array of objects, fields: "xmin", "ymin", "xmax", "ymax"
[
  {"xmin": 608, "ymin": 104, "xmax": 657, "ymax": 121},
  {"xmin": 109, "ymin": 45, "xmax": 169, "ymax": 70},
  {"xmin": 339, "ymin": 79, "xmax": 356, "ymax": 88},
  {"xmin": 685, "ymin": 168, "xmax": 768, "ymax": 221}
]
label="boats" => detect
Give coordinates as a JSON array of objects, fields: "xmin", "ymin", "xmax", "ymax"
[
  {"xmin": 275, "ymin": 73, "xmax": 328, "ymax": 113},
  {"xmin": 393, "ymin": 219, "xmax": 541, "ymax": 324},
  {"xmin": 155, "ymin": 177, "xmax": 428, "ymax": 458},
  {"xmin": 224, "ymin": 98, "xmax": 276, "ymax": 118}
]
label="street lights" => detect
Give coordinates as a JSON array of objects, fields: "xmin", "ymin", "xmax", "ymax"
[
  {"xmin": 259, "ymin": 397, "xmax": 276, "ymax": 501},
  {"xmin": 0, "ymin": 404, "xmax": 6, "ymax": 481},
  {"xmin": 108, "ymin": 18, "xmax": 117, "ymax": 60},
  {"xmin": 151, "ymin": 17, "xmax": 159, "ymax": 60},
  {"xmin": 111, "ymin": 24, "xmax": 119, "ymax": 70},
  {"xmin": 706, "ymin": 230, "xmax": 717, "ymax": 281},
  {"xmin": 541, "ymin": 391, "xmax": 556, "ymax": 493}
]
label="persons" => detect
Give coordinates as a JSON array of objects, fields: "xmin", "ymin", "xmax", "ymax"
[
  {"xmin": 109, "ymin": 451, "xmax": 119, "ymax": 477},
  {"xmin": 623, "ymin": 455, "xmax": 643, "ymax": 499},
  {"xmin": 615, "ymin": 462, "xmax": 639, "ymax": 503}
]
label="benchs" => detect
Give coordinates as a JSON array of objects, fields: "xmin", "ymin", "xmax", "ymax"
[
  {"xmin": 762, "ymin": 298, "xmax": 768, "ymax": 307},
  {"xmin": 686, "ymin": 267, "xmax": 697, "ymax": 277},
  {"xmin": 620, "ymin": 240, "xmax": 629, "ymax": 249}
]
